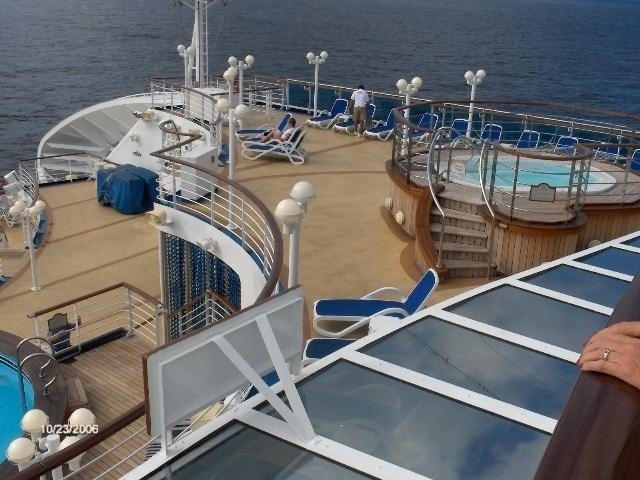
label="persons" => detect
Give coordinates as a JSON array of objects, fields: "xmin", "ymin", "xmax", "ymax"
[
  {"xmin": 575, "ymin": 319, "xmax": 640, "ymax": 392},
  {"xmin": 258, "ymin": 117, "xmax": 297, "ymax": 146},
  {"xmin": 348, "ymin": 84, "xmax": 371, "ymax": 138}
]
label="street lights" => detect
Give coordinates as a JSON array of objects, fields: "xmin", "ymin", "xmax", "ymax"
[
  {"xmin": 463, "ymin": 67, "xmax": 486, "ymax": 141},
  {"xmin": 306, "ymin": 51, "xmax": 329, "ymax": 118},
  {"xmin": 216, "ymin": 54, "xmax": 255, "ymax": 230},
  {"xmin": 176, "ymin": 43, "xmax": 195, "ymax": 120},
  {"xmin": 395, "ymin": 76, "xmax": 423, "ymax": 156},
  {"xmin": 273, "ymin": 179, "xmax": 317, "ymax": 291},
  {"xmin": 9, "ymin": 198, "xmax": 50, "ymax": 291},
  {"xmin": 4, "ymin": 407, "xmax": 98, "ymax": 480}
]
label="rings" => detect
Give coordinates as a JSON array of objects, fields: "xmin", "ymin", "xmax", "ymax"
[{"xmin": 603, "ymin": 346, "xmax": 614, "ymax": 362}]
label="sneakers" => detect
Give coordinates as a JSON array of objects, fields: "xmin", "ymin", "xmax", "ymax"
[{"xmin": 354, "ymin": 131, "xmax": 363, "ymax": 138}]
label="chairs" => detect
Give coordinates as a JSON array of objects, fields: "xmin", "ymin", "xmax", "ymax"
[
  {"xmin": 234, "ymin": 111, "xmax": 308, "ymax": 167},
  {"xmin": 302, "ymin": 266, "xmax": 445, "ymax": 369}
]
